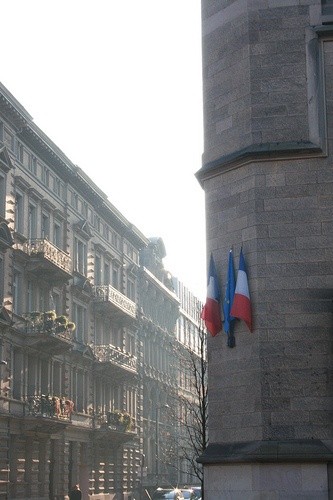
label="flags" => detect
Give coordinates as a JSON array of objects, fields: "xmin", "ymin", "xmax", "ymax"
[
  {"xmin": 229, "ymin": 248, "xmax": 253, "ymax": 334},
  {"xmin": 200, "ymin": 254, "xmax": 223, "ymax": 338},
  {"xmin": 223, "ymin": 249, "xmax": 236, "ymax": 349}
]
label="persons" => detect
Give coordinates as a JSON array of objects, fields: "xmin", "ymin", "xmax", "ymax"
[{"xmin": 70, "ymin": 485, "xmax": 81, "ymax": 500}]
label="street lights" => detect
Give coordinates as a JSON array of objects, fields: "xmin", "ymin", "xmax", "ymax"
[{"xmin": 153, "ymin": 404, "xmax": 169, "ymax": 489}]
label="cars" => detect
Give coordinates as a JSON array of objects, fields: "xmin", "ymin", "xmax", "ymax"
[
  {"xmin": 190, "ymin": 487, "xmax": 202, "ymax": 500},
  {"xmin": 179, "ymin": 489, "xmax": 198, "ymax": 500},
  {"xmin": 151, "ymin": 488, "xmax": 184, "ymax": 500}
]
